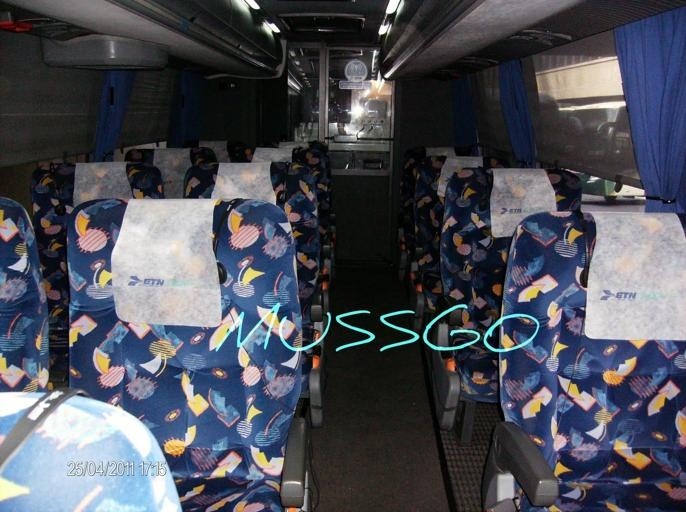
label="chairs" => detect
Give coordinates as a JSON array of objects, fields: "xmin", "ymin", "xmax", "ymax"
[
  {"xmin": 0, "ymin": 198, "xmax": 46, "ymax": 392},
  {"xmin": 124, "ymin": 147, "xmax": 230, "ymax": 200},
  {"xmin": 182, "ymin": 161, "xmax": 326, "ymax": 428},
  {"xmin": 0, "ymin": 391, "xmax": 182, "ymax": 512},
  {"xmin": 271, "ymin": 142, "xmax": 338, "ymax": 246},
  {"xmin": 29, "ymin": 163, "xmax": 161, "ymax": 388},
  {"xmin": 480, "ymin": 211, "xmax": 685, "ymax": 511},
  {"xmin": 179, "ymin": 142, "xmax": 254, "ymax": 163},
  {"xmin": 238, "ymin": 146, "xmax": 334, "ymax": 316},
  {"xmin": 399, "ymin": 145, "xmax": 455, "ymax": 273},
  {"xmin": 428, "ymin": 168, "xmax": 578, "ymax": 445},
  {"xmin": 64, "ymin": 197, "xmax": 314, "ymax": 511},
  {"xmin": 411, "ymin": 155, "xmax": 504, "ymax": 332}
]
